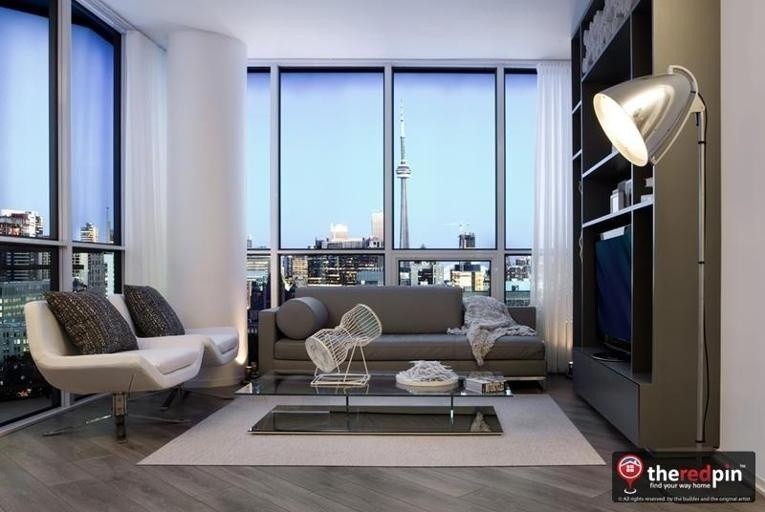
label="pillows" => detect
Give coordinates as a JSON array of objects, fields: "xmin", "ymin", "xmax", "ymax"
[
  {"xmin": 275, "ymin": 295, "xmax": 330, "ymax": 341},
  {"xmin": 41, "ymin": 286, "xmax": 140, "ymax": 356},
  {"xmin": 122, "ymin": 283, "xmax": 186, "ymax": 337}
]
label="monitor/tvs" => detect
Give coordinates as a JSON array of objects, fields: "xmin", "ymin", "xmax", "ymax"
[{"xmin": 592, "ymin": 223, "xmax": 631, "ymax": 364}]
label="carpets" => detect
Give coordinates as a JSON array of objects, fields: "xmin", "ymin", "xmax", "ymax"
[{"xmin": 134, "ymin": 391, "xmax": 608, "ymax": 467}]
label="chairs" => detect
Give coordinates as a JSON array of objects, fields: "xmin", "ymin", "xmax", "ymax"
[
  {"xmin": 106, "ymin": 292, "xmax": 240, "ymax": 411},
  {"xmin": 23, "ymin": 297, "xmax": 206, "ymax": 445}
]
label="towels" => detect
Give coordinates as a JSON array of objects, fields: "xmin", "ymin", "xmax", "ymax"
[{"xmin": 580, "ymin": 0, "xmax": 640, "ymax": 75}]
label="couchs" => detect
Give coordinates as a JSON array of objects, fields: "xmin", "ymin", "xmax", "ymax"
[{"xmin": 257, "ymin": 285, "xmax": 549, "ymax": 395}]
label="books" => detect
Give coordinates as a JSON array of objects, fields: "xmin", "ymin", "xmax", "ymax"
[
  {"xmin": 643, "ymin": 175, "xmax": 653, "ymax": 188},
  {"xmin": 640, "ymin": 193, "xmax": 653, "ymax": 204},
  {"xmin": 464, "ymin": 375, "xmax": 505, "ymax": 393}
]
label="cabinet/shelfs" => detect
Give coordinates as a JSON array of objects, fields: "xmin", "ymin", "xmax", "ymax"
[{"xmin": 570, "ymin": 0, "xmax": 721, "ymax": 460}]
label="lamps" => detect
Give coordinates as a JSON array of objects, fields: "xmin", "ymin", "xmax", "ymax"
[{"xmin": 592, "ymin": 64, "xmax": 710, "ymax": 448}]
